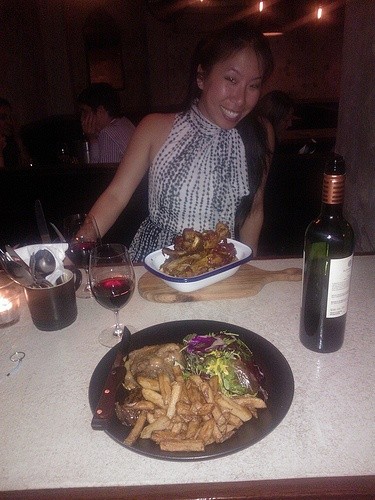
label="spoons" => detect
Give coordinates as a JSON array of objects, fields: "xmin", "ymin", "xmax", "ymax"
[
  {"xmin": 35, "ymin": 249, "xmax": 56, "ymax": 278},
  {"xmin": 5, "ymin": 261, "xmax": 42, "ymax": 289}
]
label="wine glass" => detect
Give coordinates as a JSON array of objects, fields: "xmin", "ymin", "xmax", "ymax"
[
  {"xmin": 63, "ymin": 213, "xmax": 102, "ymax": 298},
  {"xmin": 89, "ymin": 243, "xmax": 138, "ymax": 348}
]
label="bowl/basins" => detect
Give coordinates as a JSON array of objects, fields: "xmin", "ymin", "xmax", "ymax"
[{"xmin": 142, "ymin": 237, "xmax": 253, "ymax": 292}]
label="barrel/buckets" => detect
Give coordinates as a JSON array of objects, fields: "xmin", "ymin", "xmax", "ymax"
[{"xmin": 23, "ymin": 265, "xmax": 82, "ymax": 331}]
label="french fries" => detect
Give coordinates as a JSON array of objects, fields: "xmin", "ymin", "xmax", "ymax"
[{"xmin": 120, "ymin": 368, "xmax": 267, "ymax": 452}]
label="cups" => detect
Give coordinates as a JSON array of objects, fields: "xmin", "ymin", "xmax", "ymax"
[{"xmin": 0, "ymin": 269, "xmax": 21, "ymax": 328}]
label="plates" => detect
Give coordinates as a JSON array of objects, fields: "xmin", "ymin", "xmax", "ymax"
[{"xmin": 88, "ymin": 320, "xmax": 295, "ymax": 460}]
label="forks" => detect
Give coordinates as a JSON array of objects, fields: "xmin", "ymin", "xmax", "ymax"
[{"xmin": 0, "ymin": 244, "xmax": 53, "ymax": 288}]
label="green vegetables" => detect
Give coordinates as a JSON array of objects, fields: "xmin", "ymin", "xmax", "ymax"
[{"xmin": 179, "ymin": 332, "xmax": 259, "ymax": 396}]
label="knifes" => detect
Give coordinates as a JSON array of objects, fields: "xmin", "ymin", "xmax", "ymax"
[
  {"xmin": 91, "ymin": 325, "xmax": 130, "ymax": 431},
  {"xmin": 5, "ymin": 244, "xmax": 30, "ymax": 272}
]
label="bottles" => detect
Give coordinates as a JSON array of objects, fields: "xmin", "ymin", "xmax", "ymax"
[
  {"xmin": 76, "ymin": 129, "xmax": 91, "ymax": 163},
  {"xmin": 299, "ymin": 155, "xmax": 355, "ymax": 352}
]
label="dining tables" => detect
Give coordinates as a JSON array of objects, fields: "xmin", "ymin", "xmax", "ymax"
[{"xmin": 1, "ymin": 251, "xmax": 374, "ymax": 500}]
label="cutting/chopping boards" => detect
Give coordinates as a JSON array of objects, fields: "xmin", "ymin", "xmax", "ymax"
[{"xmin": 138, "ymin": 263, "xmax": 303, "ymax": 302}]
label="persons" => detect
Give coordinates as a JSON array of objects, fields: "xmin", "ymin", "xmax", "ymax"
[
  {"xmin": 0, "ymin": 96, "xmax": 32, "ymax": 171},
  {"xmin": 257, "ymin": 90, "xmax": 296, "ymax": 140},
  {"xmin": 72, "ymin": 20, "xmax": 274, "ymax": 258},
  {"xmin": 77, "ymin": 82, "xmax": 135, "ymax": 163}
]
